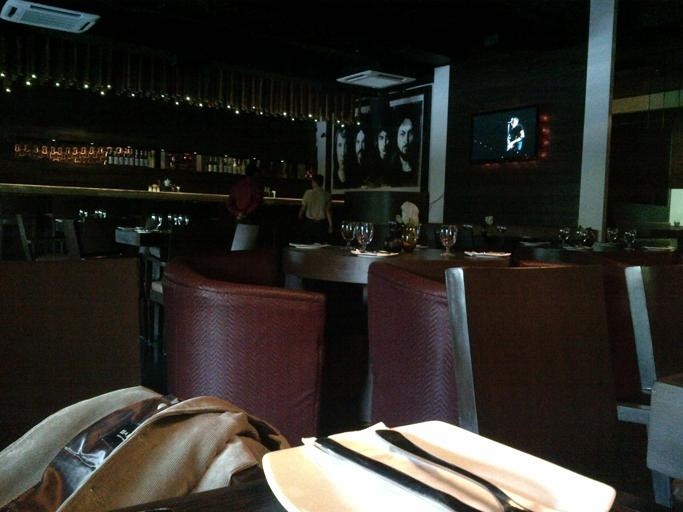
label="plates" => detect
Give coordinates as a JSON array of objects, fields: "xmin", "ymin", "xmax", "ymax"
[
  {"xmin": 464, "ymin": 251, "xmax": 512, "ymax": 257},
  {"xmin": 260, "ymin": 419, "xmax": 617, "ymax": 511}
]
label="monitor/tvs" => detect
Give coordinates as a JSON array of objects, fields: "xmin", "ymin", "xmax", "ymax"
[{"xmin": 471, "ymin": 103, "xmax": 539, "ymax": 165}]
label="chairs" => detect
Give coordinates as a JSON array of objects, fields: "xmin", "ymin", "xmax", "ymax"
[
  {"xmin": 162, "ymin": 255, "xmax": 324, "ymax": 443},
  {"xmin": 368, "ymin": 260, "xmax": 457, "ymax": 428},
  {"xmin": 625, "ymin": 263, "xmax": 680, "ymax": 507},
  {"xmin": 445, "ymin": 262, "xmax": 642, "ymax": 500},
  {"xmin": 351, "ymin": 222, "xmax": 574, "ymax": 241}
]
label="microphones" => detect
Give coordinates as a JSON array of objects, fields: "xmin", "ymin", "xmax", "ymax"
[{"xmin": 507, "ymin": 121, "xmax": 512, "ymax": 123}]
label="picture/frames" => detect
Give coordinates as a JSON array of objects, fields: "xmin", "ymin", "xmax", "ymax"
[{"xmin": 323, "ymin": 86, "xmax": 430, "ymax": 201}]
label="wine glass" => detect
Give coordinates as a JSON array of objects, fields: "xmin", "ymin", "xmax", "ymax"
[
  {"xmin": 339, "ymin": 219, "xmax": 374, "ymax": 252},
  {"xmin": 143, "ymin": 214, "xmax": 189, "ymax": 233},
  {"xmin": 558, "ymin": 226, "xmax": 637, "ymax": 250},
  {"xmin": 76, "ymin": 207, "xmax": 106, "ymax": 223}
]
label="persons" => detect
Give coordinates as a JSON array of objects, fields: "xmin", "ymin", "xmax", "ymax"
[
  {"xmin": 330, "ymin": 112, "xmax": 420, "ymax": 191},
  {"xmin": 396, "ymin": 199, "xmax": 420, "ymax": 228},
  {"xmin": 506, "ymin": 117, "xmax": 525, "ymax": 155},
  {"xmin": 298, "ymin": 173, "xmax": 332, "ymax": 243},
  {"xmin": 224, "ymin": 162, "xmax": 262, "ymax": 225}
]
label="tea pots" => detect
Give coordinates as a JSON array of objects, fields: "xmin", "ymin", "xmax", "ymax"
[{"xmin": 383, "ymin": 219, "xmax": 422, "ymax": 252}]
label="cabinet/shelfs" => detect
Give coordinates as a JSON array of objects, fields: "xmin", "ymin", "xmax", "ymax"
[{"xmin": 2, "ymin": 253, "xmax": 141, "ymax": 438}]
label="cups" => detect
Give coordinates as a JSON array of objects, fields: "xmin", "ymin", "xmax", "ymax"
[
  {"xmin": 461, "ymin": 224, "xmax": 510, "ymax": 235},
  {"xmin": 271, "ymin": 191, "xmax": 276, "ymax": 198}
]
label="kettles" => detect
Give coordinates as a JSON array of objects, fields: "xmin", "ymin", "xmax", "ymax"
[{"xmin": 439, "ymin": 224, "xmax": 460, "ymax": 258}]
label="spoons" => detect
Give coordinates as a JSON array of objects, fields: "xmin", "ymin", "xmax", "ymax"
[{"xmin": 373, "ymin": 429, "xmax": 530, "ymax": 511}]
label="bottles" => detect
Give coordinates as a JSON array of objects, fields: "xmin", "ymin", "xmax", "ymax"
[
  {"xmin": 147, "ymin": 184, "xmax": 160, "ymax": 193},
  {"xmin": 262, "ymin": 186, "xmax": 270, "ymax": 197},
  {"xmin": 280, "ymin": 159, "xmax": 288, "ymax": 179},
  {"xmin": 166, "ymin": 155, "xmax": 259, "ymax": 176},
  {"xmin": 105, "ymin": 148, "xmax": 155, "ymax": 169}
]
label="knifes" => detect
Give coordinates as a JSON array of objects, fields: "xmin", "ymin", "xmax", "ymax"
[{"xmin": 314, "ymin": 436, "xmax": 481, "ymax": 511}]
